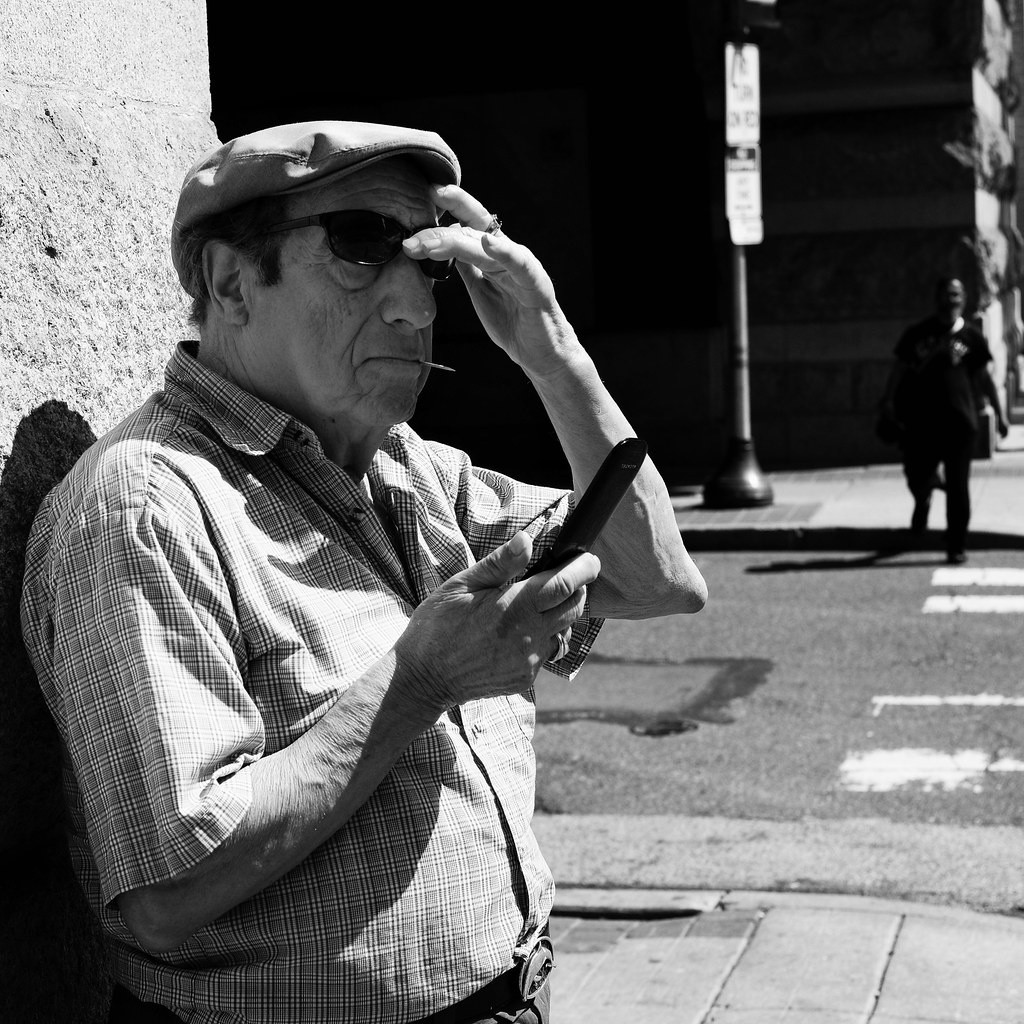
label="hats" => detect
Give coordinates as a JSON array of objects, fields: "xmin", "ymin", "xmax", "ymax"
[{"xmin": 170, "ymin": 121, "xmax": 465, "ymax": 298}]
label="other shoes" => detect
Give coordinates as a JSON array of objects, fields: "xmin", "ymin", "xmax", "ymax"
[
  {"xmin": 912, "ymin": 501, "xmax": 930, "ymax": 533},
  {"xmin": 947, "ymin": 549, "xmax": 969, "ymax": 565}
]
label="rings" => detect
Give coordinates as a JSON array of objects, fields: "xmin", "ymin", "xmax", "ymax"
[
  {"xmin": 484, "ymin": 213, "xmax": 502, "ymax": 236},
  {"xmin": 547, "ymin": 633, "xmax": 570, "ymax": 664}
]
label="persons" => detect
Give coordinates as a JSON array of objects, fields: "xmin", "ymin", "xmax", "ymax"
[
  {"xmin": 882, "ymin": 278, "xmax": 1008, "ymax": 566},
  {"xmin": 19, "ymin": 119, "xmax": 711, "ymax": 1024}
]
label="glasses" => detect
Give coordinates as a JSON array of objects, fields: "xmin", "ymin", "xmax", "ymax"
[{"xmin": 222, "ymin": 209, "xmax": 455, "ymax": 282}]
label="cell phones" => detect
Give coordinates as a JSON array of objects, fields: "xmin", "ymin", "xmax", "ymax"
[{"xmin": 519, "ymin": 438, "xmax": 650, "ymax": 582}]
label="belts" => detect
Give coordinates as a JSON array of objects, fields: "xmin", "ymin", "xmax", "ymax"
[{"xmin": 419, "ymin": 922, "xmax": 557, "ymax": 1024}]
